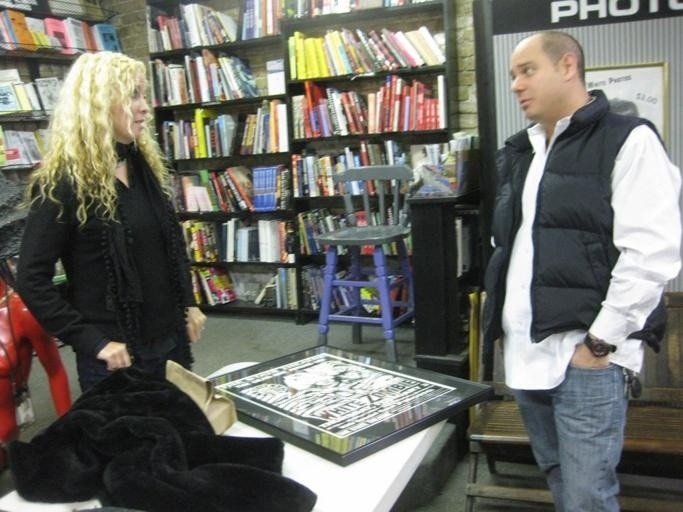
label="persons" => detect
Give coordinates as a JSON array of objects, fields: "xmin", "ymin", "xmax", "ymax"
[
  {"xmin": 473, "ymin": 30, "xmax": 682, "ymax": 511},
  {"xmin": 12, "ymin": 51, "xmax": 208, "ymax": 397}
]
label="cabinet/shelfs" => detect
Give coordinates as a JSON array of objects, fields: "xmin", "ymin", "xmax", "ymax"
[
  {"xmin": 0, "ymin": 0, "xmax": 125, "ymax": 356},
  {"xmin": 146, "ymin": 0, "xmax": 449, "ymax": 326}
]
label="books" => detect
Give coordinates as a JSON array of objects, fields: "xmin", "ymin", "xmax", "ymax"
[
  {"xmin": 178, "ymin": 217, "xmax": 295, "ymax": 263},
  {"xmin": 300, "ymin": 263, "xmax": 412, "ymax": 311},
  {"xmin": 288, "ymin": 26, "xmax": 445, "ymax": 80},
  {"xmin": 146, "ymin": 3, "xmax": 285, "ymax": 53},
  {"xmin": 286, "ymin": 3, "xmax": 416, "ymax": 18},
  {"xmin": 297, "ymin": 203, "xmax": 412, "ymax": 256},
  {"xmin": 292, "ymin": 137, "xmax": 445, "ymax": 198},
  {"xmin": 254, "ymin": 267, "xmax": 298, "ymax": 310},
  {"xmin": 169, "ymin": 164, "xmax": 291, "ymax": 213},
  {"xmin": 292, "ymin": 75, "xmax": 445, "ymax": 139},
  {"xmin": 161, "ymin": 99, "xmax": 289, "ymax": 160},
  {"xmin": 148, "ymin": 49, "xmax": 286, "ymax": 108},
  {"xmin": 0, "ymin": 0, "xmax": 124, "ymax": 173},
  {"xmin": 190, "ymin": 265, "xmax": 237, "ymax": 306}
]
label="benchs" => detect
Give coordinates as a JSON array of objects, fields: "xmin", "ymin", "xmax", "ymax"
[{"xmin": 468, "ymin": 287, "xmax": 683, "ymax": 511}]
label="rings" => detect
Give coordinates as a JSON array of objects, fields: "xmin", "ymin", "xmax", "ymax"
[{"xmin": 199, "ymin": 325, "xmax": 205, "ymax": 332}]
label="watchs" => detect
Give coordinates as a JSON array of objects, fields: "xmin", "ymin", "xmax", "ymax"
[{"xmin": 584, "ymin": 332, "xmax": 612, "ymax": 358}]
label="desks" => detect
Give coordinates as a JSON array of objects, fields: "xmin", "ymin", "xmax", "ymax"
[{"xmin": 0, "ymin": 360, "xmax": 449, "ymax": 512}]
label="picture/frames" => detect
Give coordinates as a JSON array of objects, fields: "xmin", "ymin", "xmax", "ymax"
[
  {"xmin": 584, "ymin": 60, "xmax": 671, "ymax": 160},
  {"xmin": 206, "ymin": 345, "xmax": 496, "ymax": 467}
]
